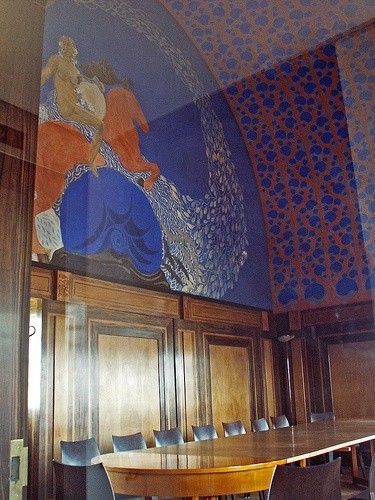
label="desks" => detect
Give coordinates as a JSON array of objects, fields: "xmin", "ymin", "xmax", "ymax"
[{"xmin": 90, "ymin": 416, "xmax": 375, "ymax": 500}]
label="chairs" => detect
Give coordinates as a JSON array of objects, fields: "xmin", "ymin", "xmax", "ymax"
[{"xmin": 52, "ymin": 413, "xmax": 375, "ymax": 500}]
